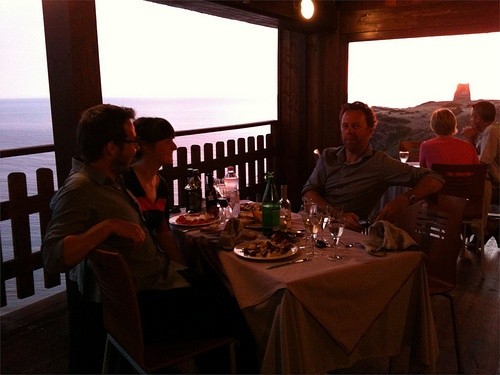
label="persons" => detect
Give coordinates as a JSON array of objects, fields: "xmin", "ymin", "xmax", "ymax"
[
  {"xmin": 301, "ymin": 102, "xmax": 445, "ymax": 232},
  {"xmin": 43, "ymin": 103, "xmax": 263, "ymax": 375},
  {"xmin": 469, "ymin": 101, "xmax": 500, "ymax": 247},
  {"xmin": 419, "ymin": 107, "xmax": 480, "ymax": 176},
  {"xmin": 119, "ymin": 117, "xmax": 185, "ymax": 266}
]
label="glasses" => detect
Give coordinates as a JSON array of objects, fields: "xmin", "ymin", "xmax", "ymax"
[{"xmin": 122, "ymin": 136, "xmax": 141, "ymax": 144}]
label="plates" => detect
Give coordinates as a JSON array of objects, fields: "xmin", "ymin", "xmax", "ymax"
[
  {"xmin": 169, "ymin": 213, "xmax": 222, "ymax": 227},
  {"xmin": 233, "ymin": 239, "xmax": 298, "ymax": 259}
]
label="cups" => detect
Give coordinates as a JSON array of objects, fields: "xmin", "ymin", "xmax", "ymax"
[{"xmin": 399, "ymin": 151, "xmax": 409, "ymax": 163}]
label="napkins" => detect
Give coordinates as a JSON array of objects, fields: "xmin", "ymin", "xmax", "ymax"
[
  {"xmin": 218, "ymin": 218, "xmax": 243, "ymax": 249},
  {"xmin": 370, "ymin": 220, "xmax": 419, "ymax": 252}
]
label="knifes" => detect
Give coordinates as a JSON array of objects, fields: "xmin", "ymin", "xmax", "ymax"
[{"xmin": 265, "ymin": 258, "xmax": 309, "ymax": 269}]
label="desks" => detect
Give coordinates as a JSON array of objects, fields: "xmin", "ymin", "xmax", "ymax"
[{"xmin": 170, "ymin": 197, "xmax": 442, "ymax": 375}]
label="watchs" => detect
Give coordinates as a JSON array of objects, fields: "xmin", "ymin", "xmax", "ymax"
[{"xmin": 404, "ymin": 189, "xmax": 415, "ymax": 205}]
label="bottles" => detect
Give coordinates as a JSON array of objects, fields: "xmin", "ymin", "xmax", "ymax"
[
  {"xmin": 205, "ymin": 172, "xmax": 217, "ymax": 213},
  {"xmin": 184, "ymin": 168, "xmax": 198, "ymax": 214},
  {"xmin": 262, "ymin": 172, "xmax": 281, "ymax": 237},
  {"xmin": 278, "ymin": 185, "xmax": 292, "ymax": 234},
  {"xmin": 224, "ymin": 166, "xmax": 241, "ymax": 218},
  {"xmin": 193, "ymin": 169, "xmax": 202, "ymax": 212}
]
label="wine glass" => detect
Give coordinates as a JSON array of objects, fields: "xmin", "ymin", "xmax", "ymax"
[
  {"xmin": 304, "ymin": 212, "xmax": 321, "ymax": 258},
  {"xmin": 326, "ymin": 216, "xmax": 345, "ymax": 261},
  {"xmin": 301, "ymin": 203, "xmax": 317, "ymax": 248}
]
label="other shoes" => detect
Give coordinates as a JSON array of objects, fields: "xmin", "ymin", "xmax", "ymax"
[{"xmin": 467, "ymin": 241, "xmax": 476, "ymax": 250}]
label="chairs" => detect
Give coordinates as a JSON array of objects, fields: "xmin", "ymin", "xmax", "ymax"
[
  {"xmin": 63, "ymin": 243, "xmax": 241, "ymax": 375},
  {"xmin": 404, "ymin": 162, "xmax": 492, "ymax": 374}
]
